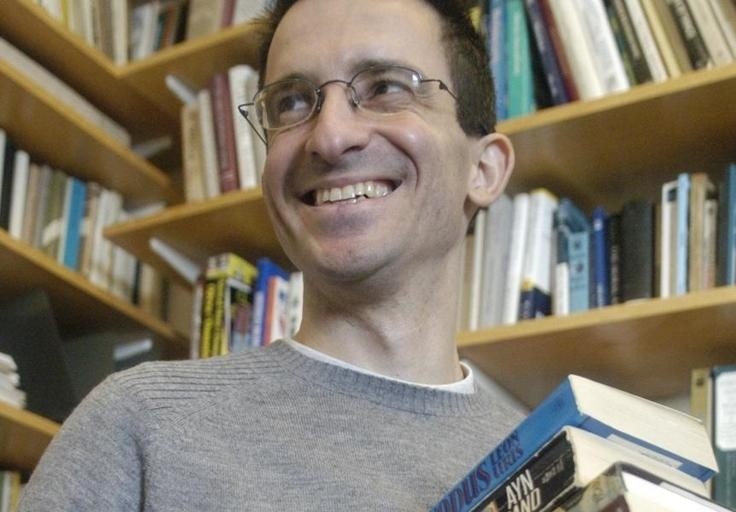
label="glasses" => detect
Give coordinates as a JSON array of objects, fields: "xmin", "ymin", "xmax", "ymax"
[{"xmin": 238, "ymin": 65, "xmax": 491, "ymax": 134}]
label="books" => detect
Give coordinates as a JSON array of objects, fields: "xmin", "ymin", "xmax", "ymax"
[
  {"xmin": 459, "ymin": 162, "xmax": 736, "ymax": 332},
  {"xmin": 181, "ymin": 65, "xmax": 266, "ymax": 204},
  {"xmin": 470, "ymin": 0, "xmax": 736, "ymax": 126},
  {"xmin": 0, "ymin": 38, "xmax": 130, "ymax": 145},
  {"xmin": 1, "ymin": 353, "xmax": 26, "ymax": 510},
  {"xmin": 1, "ymin": 129, "xmax": 304, "ymax": 360},
  {"xmin": 38, "ymin": 1, "xmax": 274, "ymax": 67},
  {"xmin": 434, "ymin": 364, "xmax": 736, "ymax": 511}
]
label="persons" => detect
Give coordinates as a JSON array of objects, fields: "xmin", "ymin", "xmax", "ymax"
[{"xmin": 27, "ymin": 1, "xmax": 529, "ymax": 510}]
